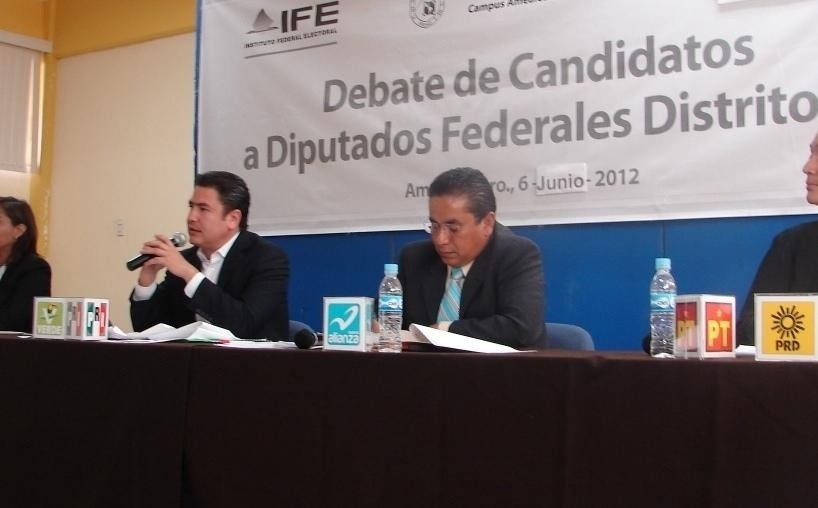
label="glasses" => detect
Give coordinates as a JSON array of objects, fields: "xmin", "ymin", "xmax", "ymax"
[{"xmin": 423, "ymin": 221, "xmax": 462, "ymax": 234}]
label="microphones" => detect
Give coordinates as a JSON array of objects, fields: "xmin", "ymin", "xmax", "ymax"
[
  {"xmin": 294, "ymin": 329, "xmax": 325, "ymax": 349},
  {"xmin": 642, "ymin": 333, "xmax": 673, "ymax": 354},
  {"xmin": 127, "ymin": 232, "xmax": 187, "ymax": 270}
]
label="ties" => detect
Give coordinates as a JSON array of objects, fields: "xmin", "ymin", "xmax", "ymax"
[{"xmin": 436, "ymin": 267, "xmax": 464, "ymax": 322}]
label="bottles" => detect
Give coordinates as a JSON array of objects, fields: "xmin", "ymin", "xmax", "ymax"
[
  {"xmin": 647, "ymin": 257, "xmax": 678, "ymax": 358},
  {"xmin": 376, "ymin": 263, "xmax": 406, "ymax": 353}
]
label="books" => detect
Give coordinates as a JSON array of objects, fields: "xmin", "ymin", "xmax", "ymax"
[{"xmin": 368, "ymin": 321, "xmax": 541, "ymax": 354}]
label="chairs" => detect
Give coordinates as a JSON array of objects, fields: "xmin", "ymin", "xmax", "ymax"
[
  {"xmin": 287, "ymin": 321, "xmax": 319, "ymax": 340},
  {"xmin": 546, "ymin": 324, "xmax": 595, "ymax": 351}
]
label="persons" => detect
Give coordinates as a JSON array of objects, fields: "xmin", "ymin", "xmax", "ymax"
[
  {"xmin": 128, "ymin": 170, "xmax": 292, "ymax": 342},
  {"xmin": 640, "ymin": 133, "xmax": 816, "ymax": 357},
  {"xmin": 368, "ymin": 166, "xmax": 553, "ymax": 349},
  {"xmin": 0, "ymin": 195, "xmax": 52, "ymax": 334}
]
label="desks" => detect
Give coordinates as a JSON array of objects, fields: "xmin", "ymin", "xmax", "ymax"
[{"xmin": 1, "ymin": 334, "xmax": 816, "ymax": 508}]
